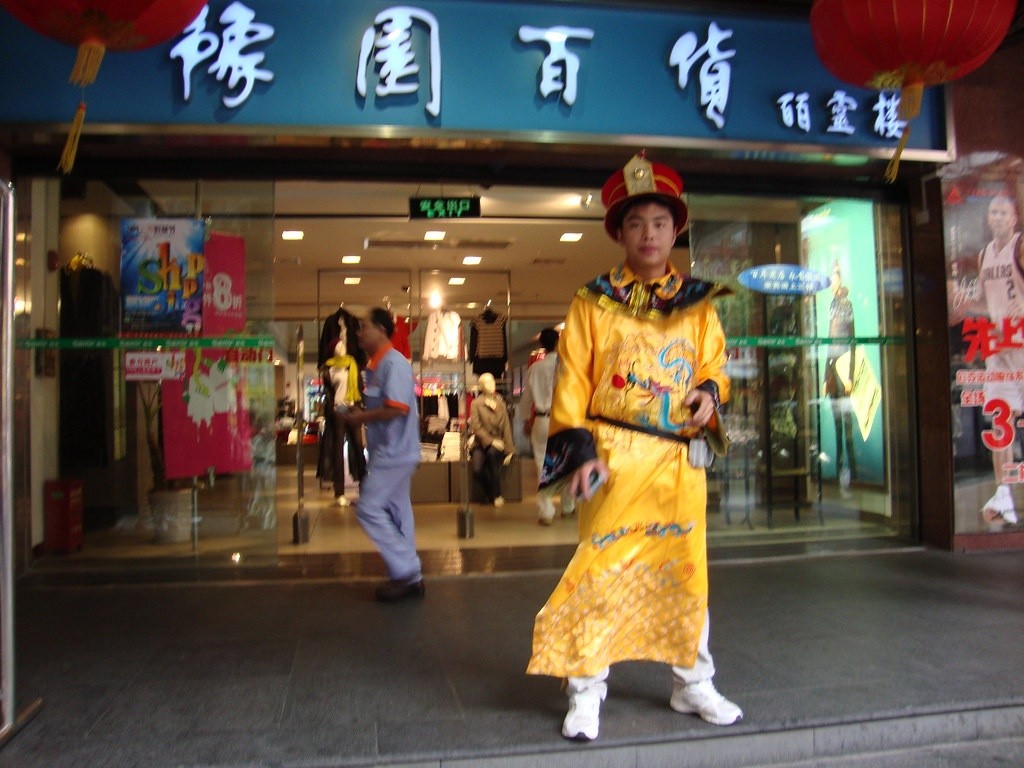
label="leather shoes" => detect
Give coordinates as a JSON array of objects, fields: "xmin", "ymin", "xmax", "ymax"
[{"xmin": 374, "ymin": 573, "xmax": 426, "ymax": 602}]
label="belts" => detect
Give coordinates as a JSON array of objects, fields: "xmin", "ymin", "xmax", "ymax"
[{"xmin": 534, "ymin": 412, "xmax": 551, "ymax": 417}]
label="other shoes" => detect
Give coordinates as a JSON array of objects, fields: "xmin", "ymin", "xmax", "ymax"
[
  {"xmin": 561, "ymin": 508, "xmax": 577, "ymax": 518},
  {"xmin": 537, "ymin": 516, "xmax": 554, "ymax": 526}
]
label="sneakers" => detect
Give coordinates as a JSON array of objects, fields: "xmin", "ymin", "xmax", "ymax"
[
  {"xmin": 982, "ymin": 485, "xmax": 1017, "ymax": 525},
  {"xmin": 669, "ymin": 676, "xmax": 744, "ymax": 726},
  {"xmin": 560, "ymin": 688, "xmax": 602, "ymax": 742}
]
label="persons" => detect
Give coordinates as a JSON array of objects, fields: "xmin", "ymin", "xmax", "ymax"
[
  {"xmin": 470, "ymin": 372, "xmax": 514, "ymax": 507},
  {"xmin": 977, "ymin": 192, "xmax": 1024, "ymax": 527},
  {"xmin": 519, "ymin": 328, "xmax": 576, "ymax": 525},
  {"xmin": 321, "ymin": 341, "xmax": 369, "ymax": 505},
  {"xmin": 536, "ymin": 152, "xmax": 744, "ymax": 738},
  {"xmin": 822, "ymin": 261, "xmax": 856, "ymax": 493},
  {"xmin": 339, "ymin": 305, "xmax": 426, "ymax": 604}
]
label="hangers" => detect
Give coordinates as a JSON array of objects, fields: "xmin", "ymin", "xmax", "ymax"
[
  {"xmin": 415, "ymin": 380, "xmax": 470, "ymax": 395},
  {"xmin": 481, "ymin": 304, "xmax": 501, "ymax": 318}
]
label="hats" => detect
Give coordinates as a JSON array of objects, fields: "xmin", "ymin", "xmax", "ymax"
[
  {"xmin": 599, "ymin": 148, "xmax": 690, "ymax": 242},
  {"xmin": 537, "ymin": 326, "xmax": 560, "ymax": 347}
]
